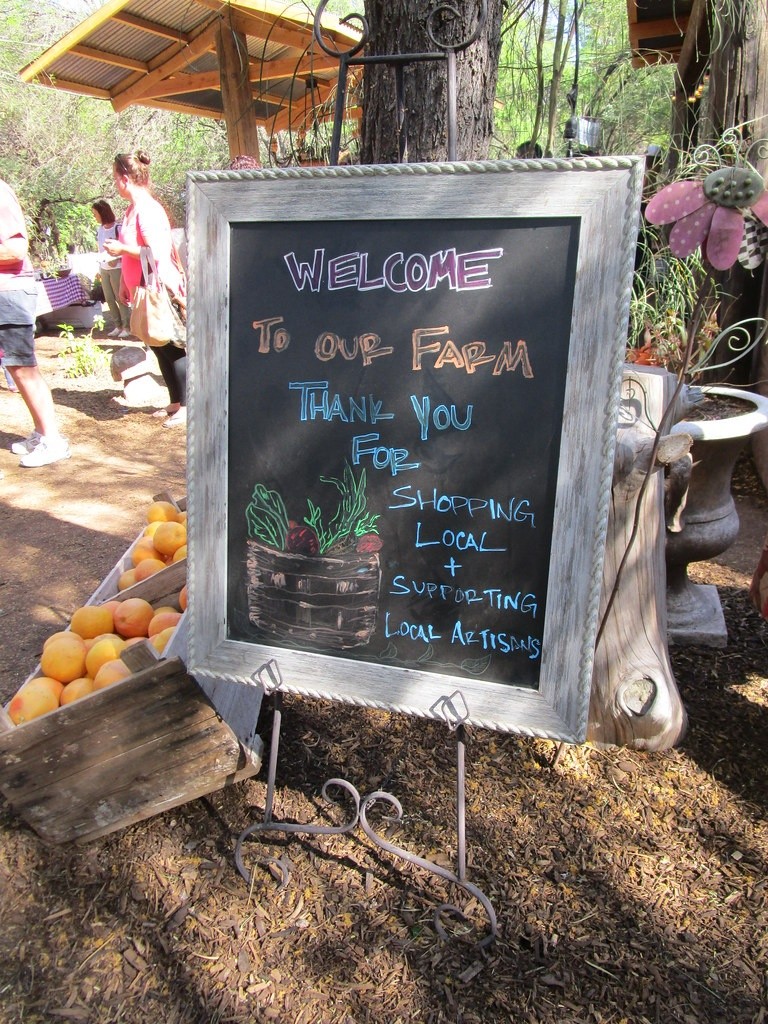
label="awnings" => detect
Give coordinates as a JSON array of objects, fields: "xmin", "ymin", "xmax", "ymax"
[
  {"xmin": 15, "ymin": 0, "xmax": 366, "ymax": 173},
  {"xmin": 626, "ymin": 0, "xmax": 695, "ymax": 69}
]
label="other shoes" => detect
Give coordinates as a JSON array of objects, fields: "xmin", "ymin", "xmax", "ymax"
[
  {"xmin": 108, "ymin": 327, "xmax": 122, "ymax": 337},
  {"xmin": 152, "ymin": 403, "xmax": 181, "ymax": 418},
  {"xmin": 8, "ymin": 386, "xmax": 20, "ymax": 393},
  {"xmin": 118, "ymin": 329, "xmax": 131, "ymax": 337},
  {"xmin": 162, "ymin": 406, "xmax": 189, "ymax": 428}
]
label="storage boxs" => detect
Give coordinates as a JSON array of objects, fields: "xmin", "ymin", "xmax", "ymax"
[
  {"xmin": 89, "ymin": 486, "xmax": 188, "ymax": 605},
  {"xmin": 0, "ymin": 557, "xmax": 265, "ymax": 847}
]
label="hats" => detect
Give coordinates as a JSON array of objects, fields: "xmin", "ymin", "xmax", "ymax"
[{"xmin": 644, "ymin": 145, "xmax": 663, "ymax": 157}]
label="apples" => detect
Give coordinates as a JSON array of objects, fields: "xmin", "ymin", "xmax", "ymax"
[
  {"xmin": 8, "ymin": 583, "xmax": 190, "ymax": 728},
  {"xmin": 116, "ymin": 500, "xmax": 188, "ymax": 591}
]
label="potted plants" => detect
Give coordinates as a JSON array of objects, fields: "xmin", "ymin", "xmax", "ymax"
[
  {"xmin": 52, "ymin": 240, "xmax": 72, "ymax": 278},
  {"xmin": 593, "ymin": 126, "xmax": 768, "ymax": 648}
]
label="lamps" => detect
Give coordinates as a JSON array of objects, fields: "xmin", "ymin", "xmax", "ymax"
[{"xmin": 269, "ymin": 52, "xmax": 361, "ymax": 167}]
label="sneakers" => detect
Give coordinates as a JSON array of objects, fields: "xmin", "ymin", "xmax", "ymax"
[
  {"xmin": 19, "ymin": 435, "xmax": 71, "ymax": 468},
  {"xmin": 11, "ymin": 429, "xmax": 42, "ymax": 455}
]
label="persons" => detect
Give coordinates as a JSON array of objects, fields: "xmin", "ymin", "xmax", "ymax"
[
  {"xmin": 515, "ymin": 141, "xmax": 665, "ymax": 296},
  {"xmin": 0, "ymin": 150, "xmax": 262, "ymax": 468}
]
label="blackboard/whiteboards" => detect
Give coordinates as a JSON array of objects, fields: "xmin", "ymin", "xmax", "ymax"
[{"xmin": 184, "ymin": 155, "xmax": 649, "ymax": 748}]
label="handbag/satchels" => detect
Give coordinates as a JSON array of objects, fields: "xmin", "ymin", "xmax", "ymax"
[{"xmin": 130, "ymin": 247, "xmax": 175, "ymax": 347}]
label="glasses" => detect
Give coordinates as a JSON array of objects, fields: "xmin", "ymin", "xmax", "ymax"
[{"xmin": 114, "ymin": 153, "xmax": 127, "ymax": 172}]
